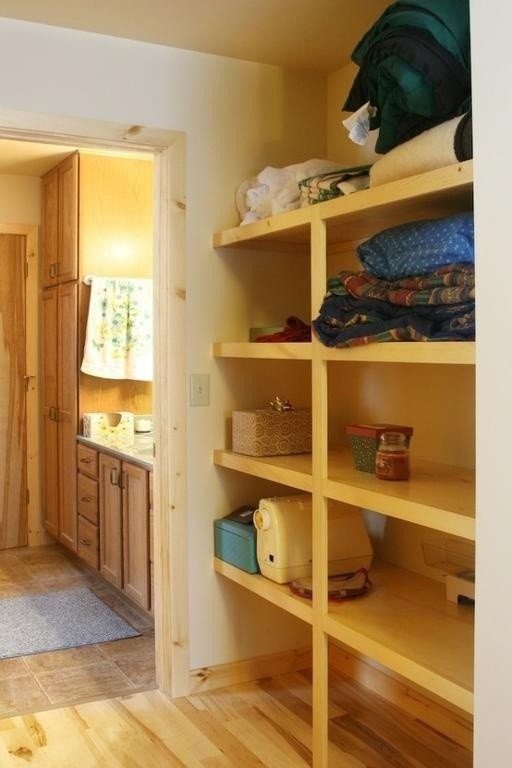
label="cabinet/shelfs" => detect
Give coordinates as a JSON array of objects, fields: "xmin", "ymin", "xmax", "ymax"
[
  {"xmin": 40, "ymin": 154, "xmax": 80, "ymax": 291},
  {"xmin": 77, "ymin": 442, "xmax": 153, "ymax": 622},
  {"xmin": 213, "ymin": 159, "xmax": 476, "ymax": 767},
  {"xmin": 38, "ymin": 281, "xmax": 78, "ymax": 556}
]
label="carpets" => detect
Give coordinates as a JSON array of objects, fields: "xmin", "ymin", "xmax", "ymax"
[{"xmin": 0, "ymin": 586, "xmax": 140, "ymax": 660}]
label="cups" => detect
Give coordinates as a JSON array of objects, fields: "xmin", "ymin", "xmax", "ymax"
[{"xmin": 134, "ymin": 415, "xmax": 152, "ymax": 433}]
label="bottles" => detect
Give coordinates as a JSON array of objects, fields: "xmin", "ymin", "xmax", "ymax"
[{"xmin": 376, "ymin": 432, "xmax": 410, "ymax": 482}]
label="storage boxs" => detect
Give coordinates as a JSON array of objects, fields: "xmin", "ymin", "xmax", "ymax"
[
  {"xmin": 232, "ymin": 407, "xmax": 312, "ymax": 455},
  {"xmin": 213, "ymin": 520, "xmax": 259, "ymax": 574}
]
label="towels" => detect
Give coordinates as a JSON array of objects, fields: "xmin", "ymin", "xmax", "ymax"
[{"xmin": 81, "ymin": 278, "xmax": 152, "ymax": 383}]
